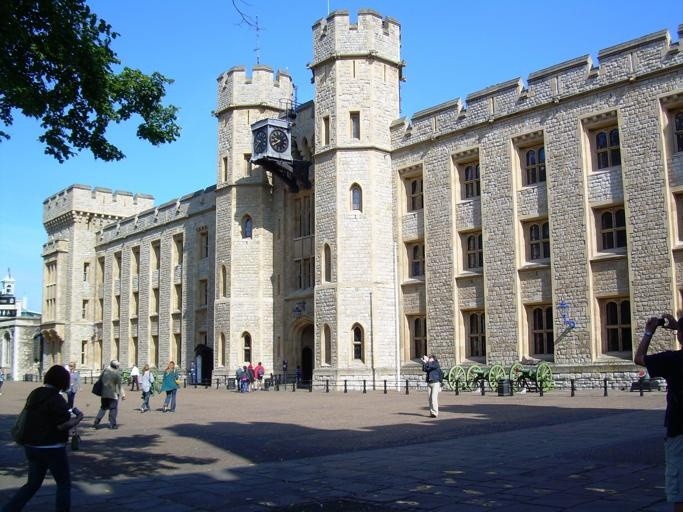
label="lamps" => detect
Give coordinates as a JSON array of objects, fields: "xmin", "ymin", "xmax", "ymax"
[{"xmin": 295, "ymin": 301, "xmax": 306, "ymax": 319}]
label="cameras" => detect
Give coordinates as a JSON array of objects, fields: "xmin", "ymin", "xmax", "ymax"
[
  {"xmin": 658, "ymin": 319, "xmax": 665, "ymax": 326},
  {"xmin": 72, "ymin": 408, "xmax": 80, "ymax": 415}
]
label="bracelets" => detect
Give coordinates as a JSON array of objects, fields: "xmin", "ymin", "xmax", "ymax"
[{"xmin": 645, "ymin": 332, "xmax": 652, "ymax": 336}]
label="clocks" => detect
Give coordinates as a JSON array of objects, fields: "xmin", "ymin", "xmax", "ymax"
[
  {"xmin": 269, "ymin": 130, "xmax": 289, "ymax": 153},
  {"xmin": 254, "ymin": 131, "xmax": 267, "ymax": 154}
]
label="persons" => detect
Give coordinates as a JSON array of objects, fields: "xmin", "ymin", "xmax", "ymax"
[
  {"xmin": 0, "ymin": 364, "xmax": 84, "ymax": 512},
  {"xmin": 236, "ymin": 362, "xmax": 303, "ymax": 393},
  {"xmin": 421, "ymin": 354, "xmax": 441, "ymax": 417},
  {"xmin": 633, "ymin": 313, "xmax": 683, "ymax": 512},
  {"xmin": 64, "ymin": 360, "xmax": 197, "ymax": 430},
  {"xmin": 0, "ymin": 367, "xmax": 4, "ymax": 396}
]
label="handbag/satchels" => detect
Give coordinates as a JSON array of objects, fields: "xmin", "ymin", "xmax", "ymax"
[
  {"xmin": 175, "ymin": 380, "xmax": 182, "ymax": 385},
  {"xmin": 149, "ymin": 386, "xmax": 154, "ymax": 394},
  {"xmin": 92, "ymin": 380, "xmax": 103, "ymax": 396},
  {"xmin": 12, "ymin": 407, "xmax": 28, "ymax": 446}
]
